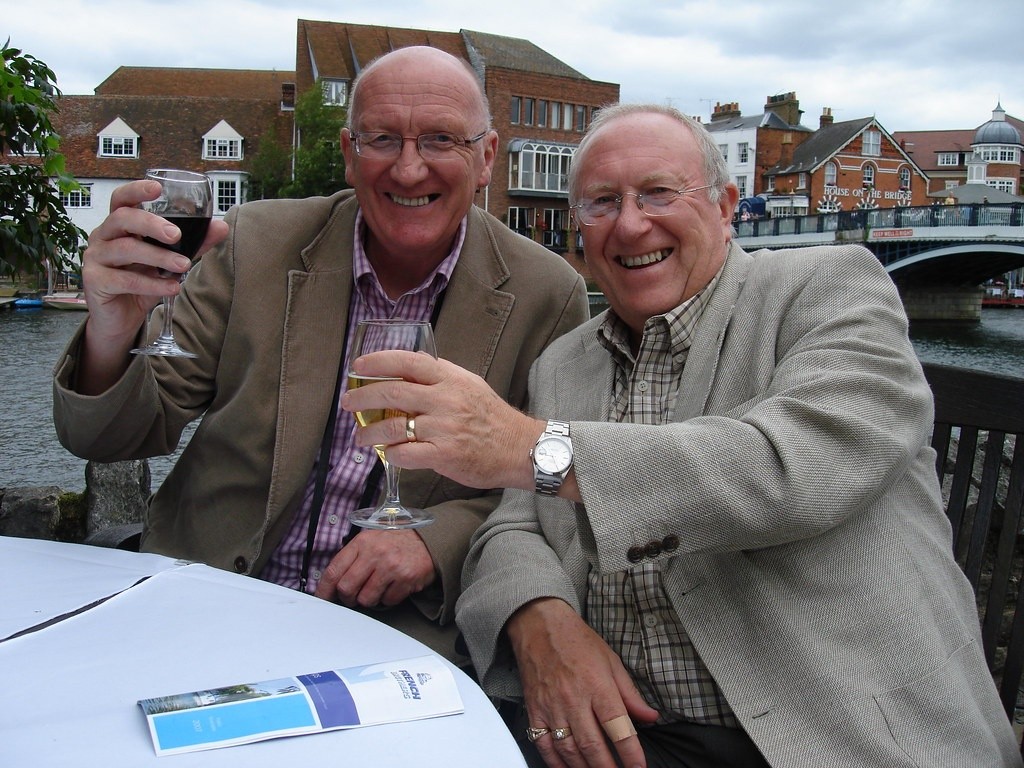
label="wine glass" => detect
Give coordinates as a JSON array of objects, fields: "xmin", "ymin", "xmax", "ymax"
[
  {"xmin": 129, "ymin": 169, "xmax": 214, "ymax": 358},
  {"xmin": 348, "ymin": 320, "xmax": 438, "ymax": 530}
]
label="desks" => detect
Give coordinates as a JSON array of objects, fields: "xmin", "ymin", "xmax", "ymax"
[{"xmin": 0, "ymin": 534, "xmax": 527, "ymax": 768}]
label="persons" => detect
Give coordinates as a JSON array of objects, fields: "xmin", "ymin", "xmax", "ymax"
[
  {"xmin": 50, "ymin": 45, "xmax": 593, "ymax": 663},
  {"xmin": 342, "ymin": 103, "xmax": 1024, "ymax": 768}
]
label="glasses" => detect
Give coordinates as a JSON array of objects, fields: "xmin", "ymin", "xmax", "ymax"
[
  {"xmin": 349, "ymin": 130, "xmax": 488, "ymax": 163},
  {"xmin": 568, "ymin": 181, "xmax": 721, "ymax": 226}
]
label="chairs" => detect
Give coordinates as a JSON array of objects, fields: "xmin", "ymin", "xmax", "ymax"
[{"xmin": 917, "ymin": 359, "xmax": 1024, "ymax": 755}]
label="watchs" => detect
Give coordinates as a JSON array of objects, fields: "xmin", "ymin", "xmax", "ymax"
[{"xmin": 528, "ymin": 419, "xmax": 575, "ymax": 497}]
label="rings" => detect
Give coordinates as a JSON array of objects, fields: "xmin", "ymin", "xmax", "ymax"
[
  {"xmin": 526, "ymin": 726, "xmax": 548, "ymax": 741},
  {"xmin": 551, "ymin": 727, "xmax": 571, "ymax": 740},
  {"xmin": 406, "ymin": 414, "xmax": 417, "ymax": 441}
]
